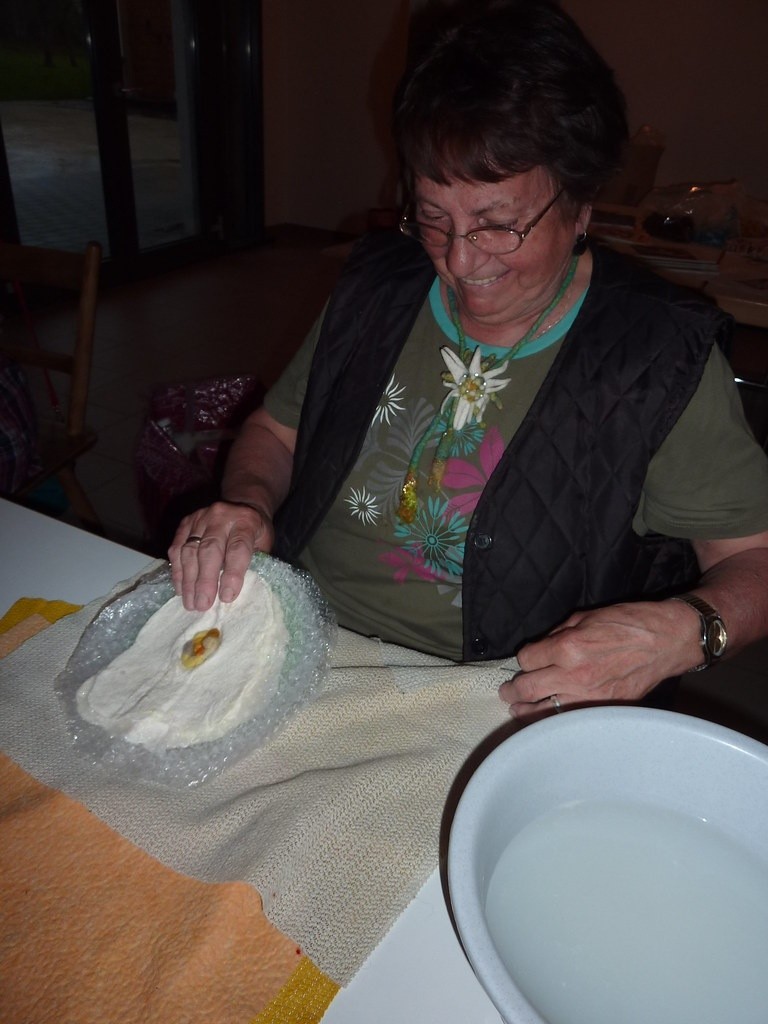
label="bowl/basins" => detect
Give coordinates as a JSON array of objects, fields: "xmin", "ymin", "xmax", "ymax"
[{"xmin": 447, "ymin": 707, "xmax": 768, "ymax": 1024}]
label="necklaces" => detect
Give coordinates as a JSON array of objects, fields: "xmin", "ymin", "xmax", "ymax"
[{"xmin": 399, "ymin": 250, "xmax": 580, "ymax": 523}]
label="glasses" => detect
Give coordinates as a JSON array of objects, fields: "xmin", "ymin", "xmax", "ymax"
[{"xmin": 397, "ymin": 186, "xmax": 567, "ymax": 255}]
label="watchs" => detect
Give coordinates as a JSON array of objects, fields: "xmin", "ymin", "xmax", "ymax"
[{"xmin": 670, "ymin": 594, "xmax": 727, "ymax": 672}]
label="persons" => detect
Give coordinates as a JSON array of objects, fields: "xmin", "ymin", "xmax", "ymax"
[{"xmin": 167, "ymin": 23, "xmax": 768, "ymax": 721}]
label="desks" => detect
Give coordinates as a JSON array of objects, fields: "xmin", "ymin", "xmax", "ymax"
[
  {"xmin": 587, "ymin": 199, "xmax": 766, "ymax": 393},
  {"xmin": 0, "ymin": 500, "xmax": 497, "ymax": 1024}
]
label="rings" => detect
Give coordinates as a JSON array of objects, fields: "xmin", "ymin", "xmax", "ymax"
[
  {"xmin": 551, "ymin": 696, "xmax": 562, "ymax": 713},
  {"xmin": 187, "ymin": 537, "xmax": 201, "ymax": 543}
]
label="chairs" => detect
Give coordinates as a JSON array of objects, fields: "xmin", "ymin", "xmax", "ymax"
[{"xmin": 0, "ymin": 239, "xmax": 102, "ymax": 538}]
